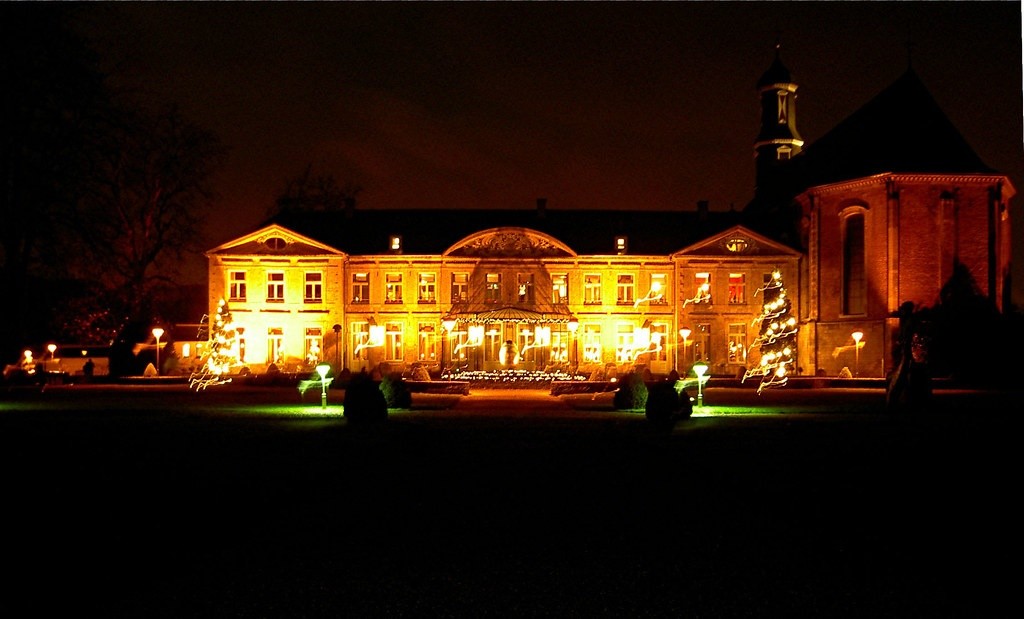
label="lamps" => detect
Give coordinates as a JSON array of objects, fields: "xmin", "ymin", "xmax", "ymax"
[
  {"xmin": 316, "ymin": 363, "xmax": 330, "ymax": 408},
  {"xmin": 692, "ymin": 361, "xmax": 708, "ymax": 408}
]
